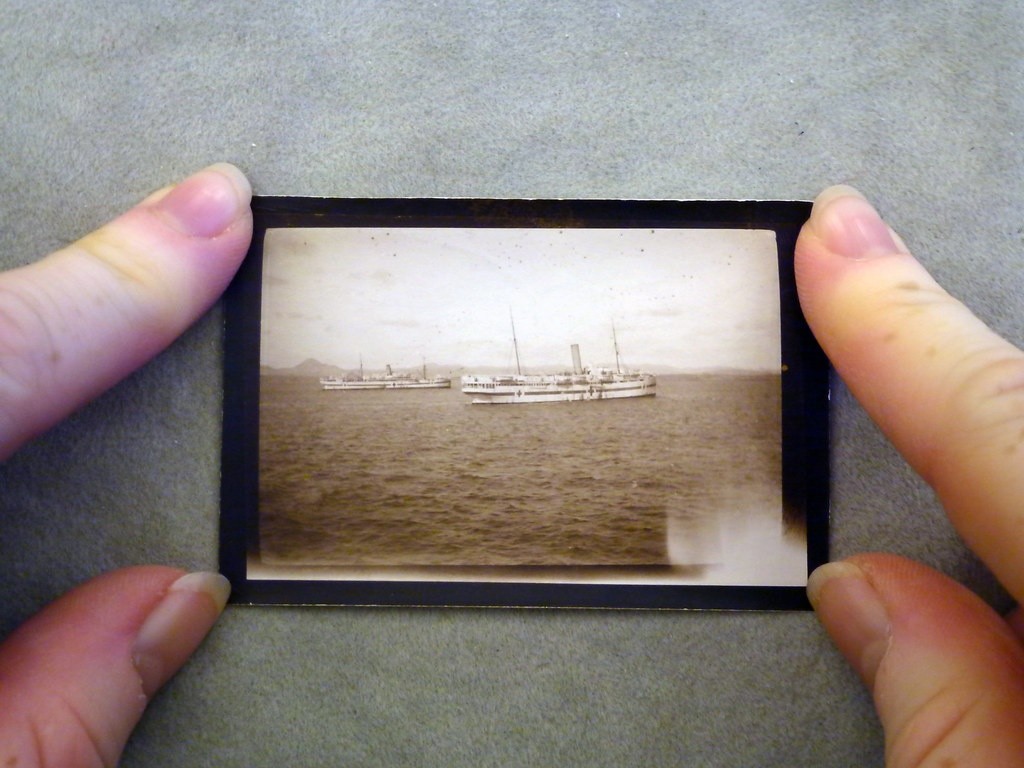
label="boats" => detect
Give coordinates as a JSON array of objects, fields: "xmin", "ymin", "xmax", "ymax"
[
  {"xmin": 319, "ymin": 362, "xmax": 451, "ymax": 391},
  {"xmin": 461, "ymin": 303, "xmax": 659, "ymax": 405}
]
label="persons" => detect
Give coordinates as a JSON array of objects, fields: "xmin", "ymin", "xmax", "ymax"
[{"xmin": 0, "ymin": 161, "xmax": 1023, "ymax": 767}]
